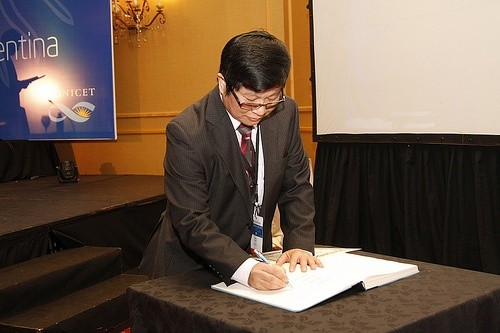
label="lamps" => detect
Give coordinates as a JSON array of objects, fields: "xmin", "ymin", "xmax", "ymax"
[
  {"xmin": 56, "ymin": 160, "xmax": 80, "ymax": 183},
  {"xmin": 112, "ymin": 0, "xmax": 167, "ymax": 48}
]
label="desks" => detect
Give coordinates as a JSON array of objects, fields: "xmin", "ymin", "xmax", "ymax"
[{"xmin": 126, "ymin": 245, "xmax": 500, "ymax": 333}]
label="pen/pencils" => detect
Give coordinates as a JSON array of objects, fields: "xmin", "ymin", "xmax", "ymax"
[{"xmin": 250, "ymin": 248, "xmax": 289, "ymax": 284}]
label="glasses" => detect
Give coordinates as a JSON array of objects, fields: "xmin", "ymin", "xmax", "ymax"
[{"xmin": 228, "ymin": 83, "xmax": 285, "ymax": 111}]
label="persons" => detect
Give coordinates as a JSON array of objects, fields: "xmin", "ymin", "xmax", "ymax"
[{"xmin": 138, "ymin": 27, "xmax": 324, "ymax": 291}]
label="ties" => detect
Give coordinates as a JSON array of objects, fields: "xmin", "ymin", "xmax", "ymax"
[{"xmin": 238, "ymin": 123, "xmax": 255, "ymax": 192}]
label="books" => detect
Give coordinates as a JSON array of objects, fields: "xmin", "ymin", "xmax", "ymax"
[{"xmin": 211, "ymin": 252, "xmax": 419, "ymax": 313}]
